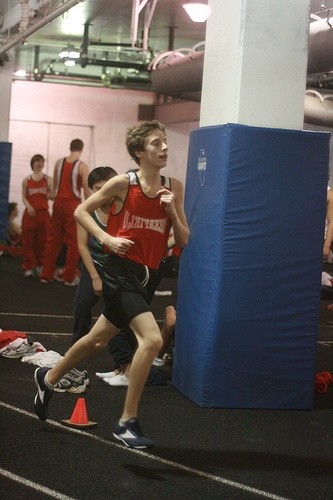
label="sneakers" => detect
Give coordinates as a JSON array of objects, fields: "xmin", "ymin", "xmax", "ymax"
[
  {"xmin": 34, "ymin": 367, "xmax": 53, "ymax": 420},
  {"xmin": 53, "ymin": 368, "xmax": 90, "ymax": 393},
  {"xmin": 113, "ymin": 416, "xmax": 149, "ymax": 449}
]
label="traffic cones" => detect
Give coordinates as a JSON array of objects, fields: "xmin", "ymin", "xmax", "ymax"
[{"xmin": 59, "ymin": 396, "xmax": 97, "ymax": 429}]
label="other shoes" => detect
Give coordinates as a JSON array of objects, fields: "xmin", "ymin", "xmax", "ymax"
[
  {"xmin": 41, "ymin": 279, "xmax": 48, "ymax": 283},
  {"xmin": 65, "ymin": 278, "xmax": 79, "ymax": 286},
  {"xmin": 2, "ymin": 341, "xmax": 46, "ymax": 358},
  {"xmin": 24, "ymin": 270, "xmax": 34, "ymax": 278}
]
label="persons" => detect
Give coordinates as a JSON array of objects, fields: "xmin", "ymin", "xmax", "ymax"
[
  {"xmin": 7, "ymin": 120, "xmax": 190, "ymax": 449},
  {"xmin": 321, "ymin": 174, "xmax": 333, "ymax": 311}
]
